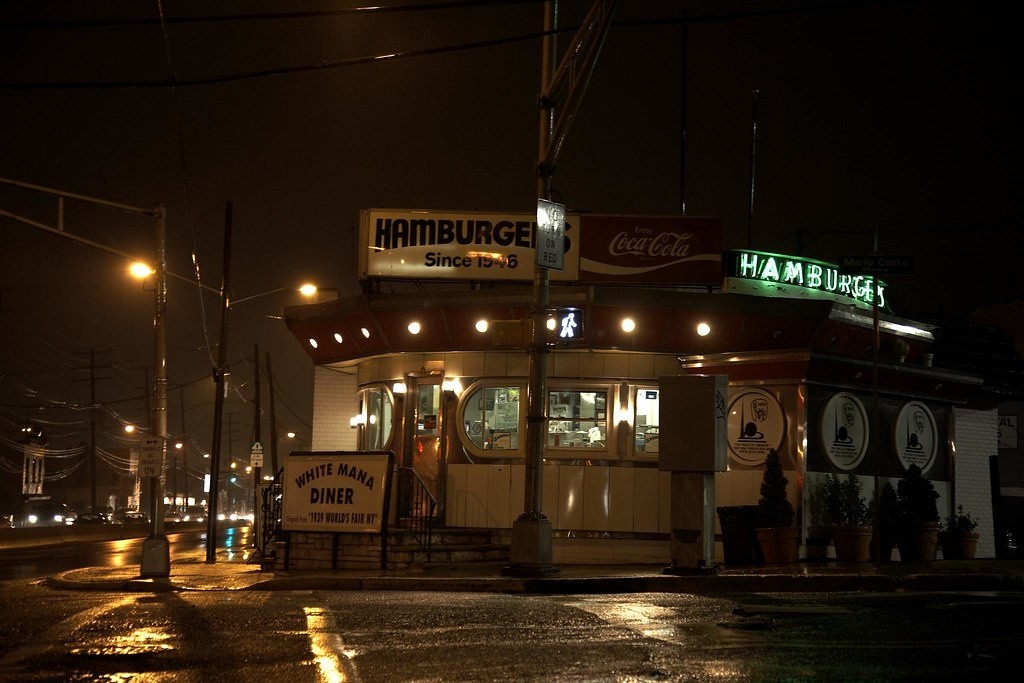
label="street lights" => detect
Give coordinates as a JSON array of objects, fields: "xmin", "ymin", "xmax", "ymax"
[{"xmin": 127, "ymin": 258, "xmax": 167, "ymax": 533}]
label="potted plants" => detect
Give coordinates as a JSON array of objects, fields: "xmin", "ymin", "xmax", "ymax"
[
  {"xmin": 892, "ymin": 462, "xmax": 941, "ymax": 563},
  {"xmin": 942, "ymin": 505, "xmax": 980, "ymax": 561},
  {"xmin": 752, "ymin": 448, "xmax": 801, "ymax": 566},
  {"xmin": 806, "ymin": 467, "xmax": 874, "ymax": 566}
]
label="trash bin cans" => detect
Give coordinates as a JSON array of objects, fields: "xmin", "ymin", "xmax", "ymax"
[{"xmin": 717, "ymin": 505, "xmax": 793, "ymax": 568}]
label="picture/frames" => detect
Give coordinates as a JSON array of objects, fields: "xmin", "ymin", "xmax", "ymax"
[{"xmin": 479, "ymin": 398, "xmax": 494, "ymax": 410}]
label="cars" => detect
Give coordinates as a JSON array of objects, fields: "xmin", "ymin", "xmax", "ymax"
[{"xmin": 0, "ymin": 505, "xmax": 207, "ymax": 528}]
